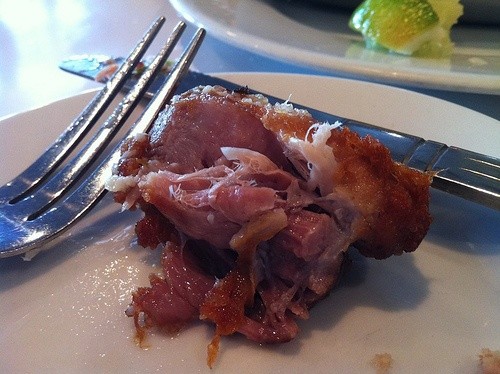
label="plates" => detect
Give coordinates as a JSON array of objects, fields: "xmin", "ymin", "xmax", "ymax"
[
  {"xmin": 168, "ymin": 2, "xmax": 499, "ymax": 101},
  {"xmin": 3, "ymin": 70, "xmax": 499, "ymax": 374}
]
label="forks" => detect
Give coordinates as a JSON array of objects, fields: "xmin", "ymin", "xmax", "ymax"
[{"xmin": 1, "ymin": 17, "xmax": 210, "ymax": 260}]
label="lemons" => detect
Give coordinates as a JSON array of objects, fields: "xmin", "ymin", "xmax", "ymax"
[{"xmin": 349, "ymin": 0, "xmax": 464, "ymax": 58}]
